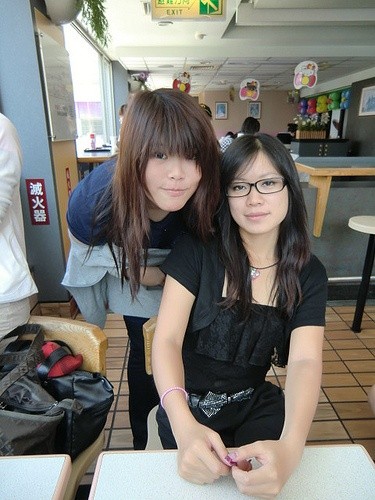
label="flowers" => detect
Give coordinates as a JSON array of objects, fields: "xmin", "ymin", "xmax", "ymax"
[{"xmin": 294, "ymin": 112, "xmax": 330, "ymax": 128}]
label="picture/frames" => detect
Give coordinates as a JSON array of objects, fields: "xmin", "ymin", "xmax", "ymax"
[
  {"xmin": 247, "ymin": 101, "xmax": 262, "ymax": 120},
  {"xmin": 215, "ymin": 101, "xmax": 229, "ymax": 120}
]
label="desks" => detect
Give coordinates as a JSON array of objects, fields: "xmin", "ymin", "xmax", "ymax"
[
  {"xmin": 77, "ymin": 145, "xmax": 115, "ymax": 174},
  {"xmin": 293, "ymin": 157, "xmax": 375, "ymax": 237},
  {"xmin": 88, "ymin": 444, "xmax": 375, "ymax": 500},
  {"xmin": 0, "ymin": 454, "xmax": 71, "ymax": 500}
]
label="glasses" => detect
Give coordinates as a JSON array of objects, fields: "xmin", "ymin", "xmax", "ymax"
[{"xmin": 225, "ymin": 177, "xmax": 287, "ymax": 197}]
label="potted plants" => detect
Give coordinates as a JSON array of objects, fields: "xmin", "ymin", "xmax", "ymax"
[{"xmin": 296, "ymin": 130, "xmax": 326, "ymax": 139}]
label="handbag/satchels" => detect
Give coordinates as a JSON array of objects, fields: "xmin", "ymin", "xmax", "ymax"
[
  {"xmin": 0, "ymin": 324, "xmax": 66, "ymax": 457},
  {"xmin": 0, "ymin": 340, "xmax": 115, "ymax": 461}
]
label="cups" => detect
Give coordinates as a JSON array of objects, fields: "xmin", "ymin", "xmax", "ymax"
[{"xmin": 110, "ymin": 136, "xmax": 118, "ymax": 153}]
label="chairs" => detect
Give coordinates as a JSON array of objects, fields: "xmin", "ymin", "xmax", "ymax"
[{"xmin": 20, "ymin": 316, "xmax": 108, "ymax": 500}]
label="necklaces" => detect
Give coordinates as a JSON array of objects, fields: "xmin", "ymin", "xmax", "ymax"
[{"xmin": 248, "ymin": 261, "xmax": 280, "ymax": 279}]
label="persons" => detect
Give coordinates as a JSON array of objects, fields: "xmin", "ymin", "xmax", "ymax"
[
  {"xmin": 152, "ymin": 133, "xmax": 330, "ymax": 500},
  {"xmin": 115, "ymin": 100, "xmax": 261, "ymax": 151},
  {"xmin": 65, "ymin": 89, "xmax": 221, "ymax": 451},
  {"xmin": 0, "ymin": 111, "xmax": 45, "ymax": 354}
]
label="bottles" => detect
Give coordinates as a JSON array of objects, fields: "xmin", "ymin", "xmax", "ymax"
[{"xmin": 90, "ymin": 134, "xmax": 95, "ymax": 150}]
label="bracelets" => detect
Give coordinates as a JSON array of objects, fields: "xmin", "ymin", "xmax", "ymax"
[{"xmin": 160, "ymin": 387, "xmax": 189, "ymax": 408}]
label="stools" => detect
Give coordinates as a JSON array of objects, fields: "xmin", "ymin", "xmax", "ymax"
[{"xmin": 347, "ymin": 215, "xmax": 375, "ymax": 333}]
